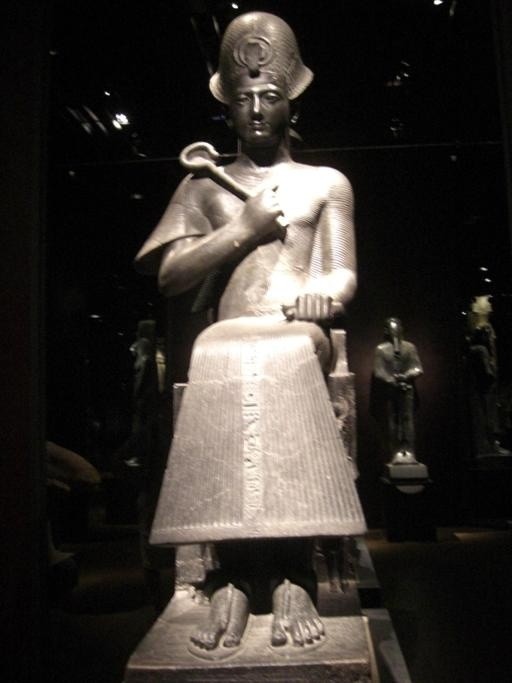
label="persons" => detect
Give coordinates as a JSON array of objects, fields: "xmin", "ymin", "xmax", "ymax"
[
  {"xmin": 464, "ymin": 295, "xmax": 505, "ymax": 452},
  {"xmin": 133, "ymin": 9, "xmax": 370, "ymax": 661},
  {"xmin": 373, "ymin": 317, "xmax": 426, "ymax": 466}
]
